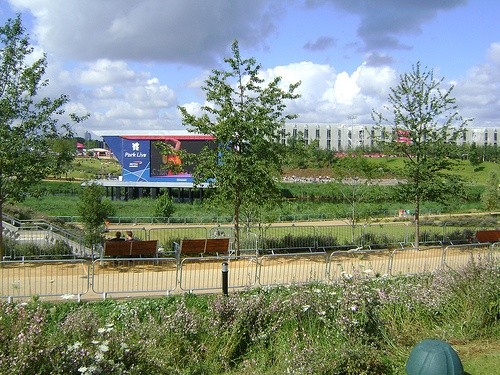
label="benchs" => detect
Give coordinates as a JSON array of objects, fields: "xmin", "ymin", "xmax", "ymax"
[
  {"xmin": 100, "ymin": 239, "xmax": 160, "ymax": 267},
  {"xmin": 171, "ymin": 238, "xmax": 230, "ymax": 267},
  {"xmin": 466, "ymin": 229, "xmax": 500, "ymax": 249}
]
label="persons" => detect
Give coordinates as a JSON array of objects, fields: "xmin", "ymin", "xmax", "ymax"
[
  {"xmin": 161, "ymin": 139, "xmax": 185, "ymax": 176},
  {"xmin": 124, "ymin": 231, "xmax": 137, "ymax": 263},
  {"xmin": 110, "ymin": 232, "xmax": 126, "ymax": 265}
]
